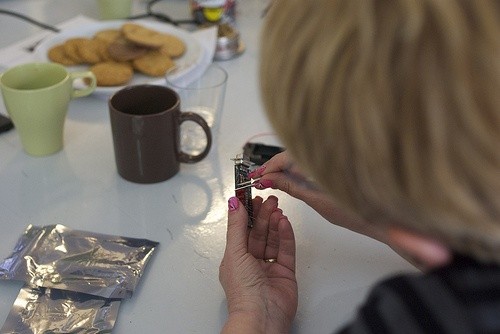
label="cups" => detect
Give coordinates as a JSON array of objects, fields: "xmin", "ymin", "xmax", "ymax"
[
  {"xmin": 0, "ymin": 61, "xmax": 97, "ymax": 157},
  {"xmin": 108, "ymin": 84, "xmax": 212, "ymax": 184},
  {"xmin": 165, "ymin": 63, "xmax": 228, "ymax": 152},
  {"xmin": 97, "ymin": 0, "xmax": 132, "ymax": 20}
]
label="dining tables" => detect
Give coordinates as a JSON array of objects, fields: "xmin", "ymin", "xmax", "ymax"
[{"xmin": 0, "ymin": 4, "xmax": 415, "ymax": 334}]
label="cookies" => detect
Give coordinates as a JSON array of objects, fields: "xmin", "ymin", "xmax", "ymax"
[{"xmin": 47, "ymin": 23, "xmax": 186, "ymax": 87}]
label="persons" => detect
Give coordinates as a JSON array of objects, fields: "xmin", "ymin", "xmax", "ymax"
[{"xmin": 218, "ymin": 0, "xmax": 500, "ymax": 334}]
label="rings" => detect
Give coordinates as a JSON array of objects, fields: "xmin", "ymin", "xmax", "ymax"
[{"xmin": 265, "ymin": 258, "xmax": 276, "ymax": 263}]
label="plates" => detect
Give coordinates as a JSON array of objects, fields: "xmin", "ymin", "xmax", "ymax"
[{"xmin": 33, "ymin": 20, "xmax": 203, "ymax": 94}]
